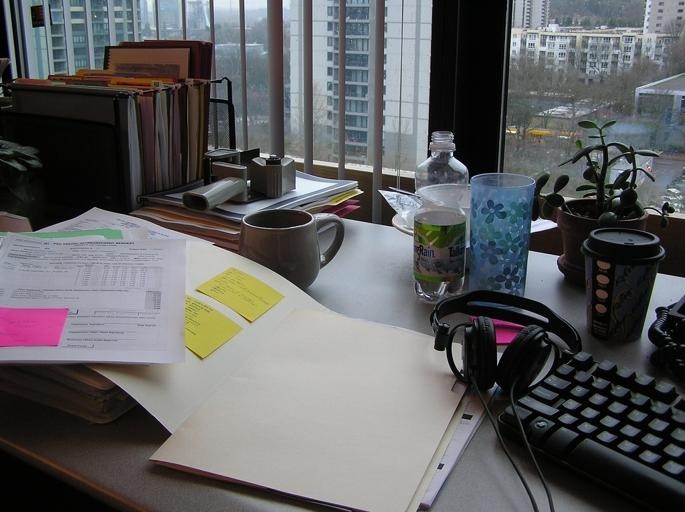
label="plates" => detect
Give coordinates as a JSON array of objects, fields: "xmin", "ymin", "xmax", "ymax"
[{"xmin": 389, "ymin": 208, "xmax": 416, "ymax": 239}]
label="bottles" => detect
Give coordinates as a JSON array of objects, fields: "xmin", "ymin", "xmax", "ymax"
[{"xmin": 411, "ymin": 132, "xmax": 470, "ymax": 302}]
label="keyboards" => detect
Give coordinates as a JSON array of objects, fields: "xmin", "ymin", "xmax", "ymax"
[{"xmin": 497, "ymin": 351, "xmax": 685, "ymax": 512}]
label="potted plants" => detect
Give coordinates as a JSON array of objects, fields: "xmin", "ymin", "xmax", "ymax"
[{"xmin": 529, "ymin": 116, "xmax": 675, "ymax": 276}]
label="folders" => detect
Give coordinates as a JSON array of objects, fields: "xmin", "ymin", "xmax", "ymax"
[{"xmin": 11, "ymin": 69, "xmax": 210, "ymax": 213}]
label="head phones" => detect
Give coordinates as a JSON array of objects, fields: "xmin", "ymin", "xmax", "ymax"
[{"xmin": 430, "ymin": 290, "xmax": 582, "ymax": 391}]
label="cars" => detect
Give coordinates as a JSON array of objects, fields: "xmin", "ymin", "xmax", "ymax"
[{"xmin": 659, "ymin": 168, "xmax": 685, "ymax": 213}]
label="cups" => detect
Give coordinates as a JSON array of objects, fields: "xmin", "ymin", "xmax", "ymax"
[
  {"xmin": 469, "ymin": 169, "xmax": 536, "ymax": 304},
  {"xmin": 416, "ymin": 183, "xmax": 474, "ymax": 239},
  {"xmin": 237, "ymin": 208, "xmax": 345, "ymax": 291},
  {"xmin": 584, "ymin": 226, "xmax": 665, "ymax": 347}
]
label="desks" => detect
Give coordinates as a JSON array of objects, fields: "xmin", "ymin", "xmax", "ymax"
[{"xmin": 0, "ymin": 205, "xmax": 684, "ymax": 509}]
